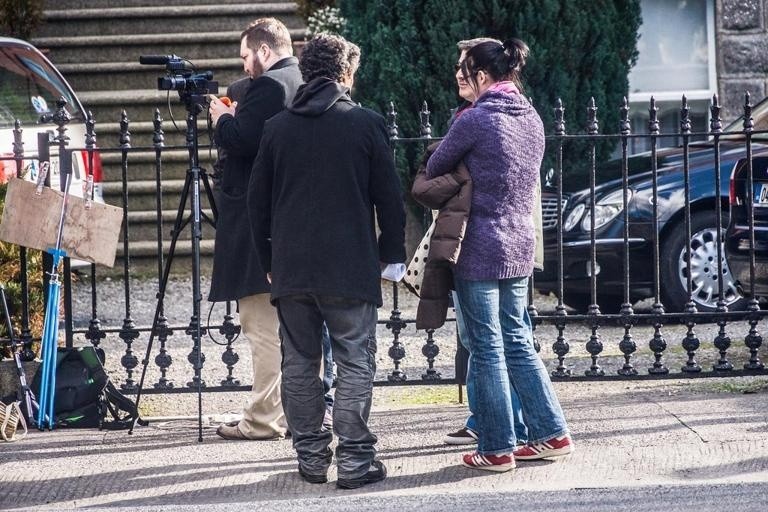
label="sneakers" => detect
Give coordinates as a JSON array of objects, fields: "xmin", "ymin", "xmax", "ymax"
[
  {"xmin": 511, "ymin": 431, "xmax": 572, "ymax": 460},
  {"xmin": 443, "ymin": 426, "xmax": 484, "ymax": 446},
  {"xmin": 297, "ymin": 458, "xmax": 329, "ymax": 484},
  {"xmin": 462, "ymin": 447, "xmax": 516, "ymax": 472},
  {"xmin": 214, "ymin": 425, "xmax": 282, "ymax": 442},
  {"xmin": 336, "ymin": 460, "xmax": 388, "ymax": 488},
  {"xmin": 224, "ymin": 419, "xmax": 247, "ymax": 429}
]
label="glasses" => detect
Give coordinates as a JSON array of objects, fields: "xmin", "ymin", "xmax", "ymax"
[{"xmin": 455, "ymin": 63, "xmax": 465, "ymax": 70}]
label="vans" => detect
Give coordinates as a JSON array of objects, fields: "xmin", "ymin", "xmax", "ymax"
[{"xmin": 0, "ymin": 37, "xmax": 104, "ymax": 269}]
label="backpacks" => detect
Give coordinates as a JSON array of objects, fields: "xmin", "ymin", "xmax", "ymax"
[{"xmin": 25, "ymin": 344, "xmax": 109, "ymax": 429}]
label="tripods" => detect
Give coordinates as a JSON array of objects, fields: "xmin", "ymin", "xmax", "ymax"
[
  {"xmin": 35, "ymin": 274, "xmax": 61, "ymax": 431},
  {"xmin": 128, "ymin": 119, "xmax": 221, "ymax": 443}
]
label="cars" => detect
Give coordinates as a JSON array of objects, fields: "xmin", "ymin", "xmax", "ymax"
[
  {"xmin": 725, "ymin": 151, "xmax": 768, "ymax": 297},
  {"xmin": 532, "ymin": 94, "xmax": 768, "ymax": 323}
]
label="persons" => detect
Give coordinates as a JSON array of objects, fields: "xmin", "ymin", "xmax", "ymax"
[
  {"xmin": 246, "ymin": 32, "xmax": 407, "ymax": 489},
  {"xmin": 442, "ymin": 36, "xmax": 531, "ymax": 447},
  {"xmin": 425, "ymin": 37, "xmax": 574, "ymax": 473},
  {"xmin": 208, "ymin": 16, "xmax": 306, "ymax": 441}
]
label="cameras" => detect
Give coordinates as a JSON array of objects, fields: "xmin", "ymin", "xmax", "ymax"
[{"xmin": 138, "ymin": 52, "xmax": 219, "ymax": 115}]
